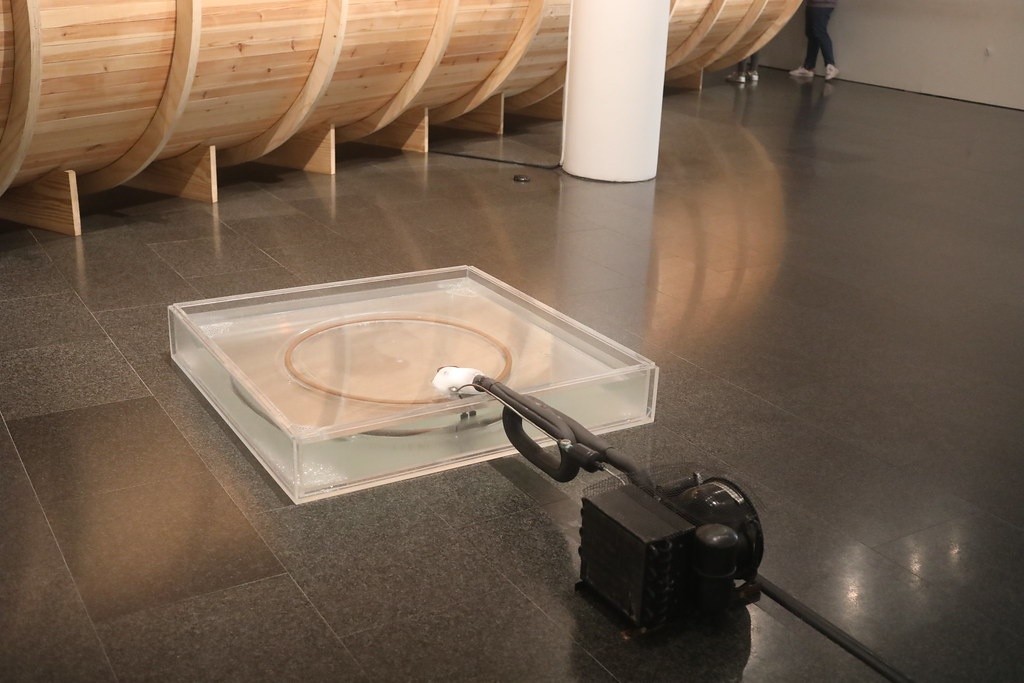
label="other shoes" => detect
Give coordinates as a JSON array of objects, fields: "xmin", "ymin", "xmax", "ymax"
[
  {"xmin": 745, "ymin": 70, "xmax": 759, "ymax": 81},
  {"xmin": 726, "ymin": 72, "xmax": 746, "ymax": 82}
]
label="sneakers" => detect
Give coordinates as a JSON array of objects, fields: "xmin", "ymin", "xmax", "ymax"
[
  {"xmin": 824, "ymin": 63, "xmax": 839, "ymax": 79},
  {"xmin": 788, "ymin": 65, "xmax": 815, "ymax": 77}
]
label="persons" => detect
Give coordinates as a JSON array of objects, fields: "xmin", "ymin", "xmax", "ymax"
[
  {"xmin": 725, "ymin": 50, "xmax": 763, "ymax": 83},
  {"xmin": 788, "ymin": 0, "xmax": 841, "ymax": 81}
]
